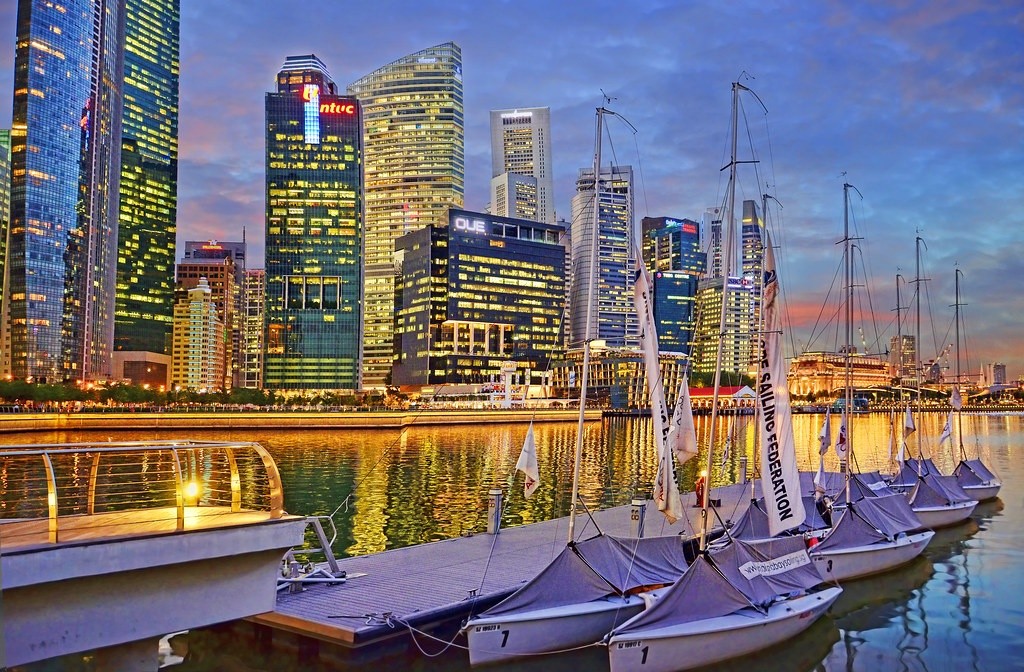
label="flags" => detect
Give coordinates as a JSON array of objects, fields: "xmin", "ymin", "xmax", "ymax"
[
  {"xmin": 814, "ymin": 404, "xmax": 831, "ymax": 500},
  {"xmin": 940, "ymin": 413, "xmax": 952, "ymax": 445},
  {"xmin": 951, "ymin": 386, "xmax": 963, "ymax": 411},
  {"xmin": 836, "ymin": 410, "xmax": 847, "ymax": 461},
  {"xmin": 515, "ymin": 425, "xmax": 540, "ymax": 499},
  {"xmin": 667, "ymin": 372, "xmax": 699, "ymax": 462},
  {"xmin": 890, "ymin": 407, "xmax": 894, "ymax": 460},
  {"xmin": 896, "ymin": 405, "xmax": 917, "ymax": 469}
]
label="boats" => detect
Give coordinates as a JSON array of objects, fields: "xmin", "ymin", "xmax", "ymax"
[{"xmin": 833, "ymin": 398, "xmax": 873, "ymax": 414}]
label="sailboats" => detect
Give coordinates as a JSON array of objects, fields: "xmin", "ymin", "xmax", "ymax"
[{"xmin": 463, "ymin": 73, "xmax": 1003, "ymax": 672}]
